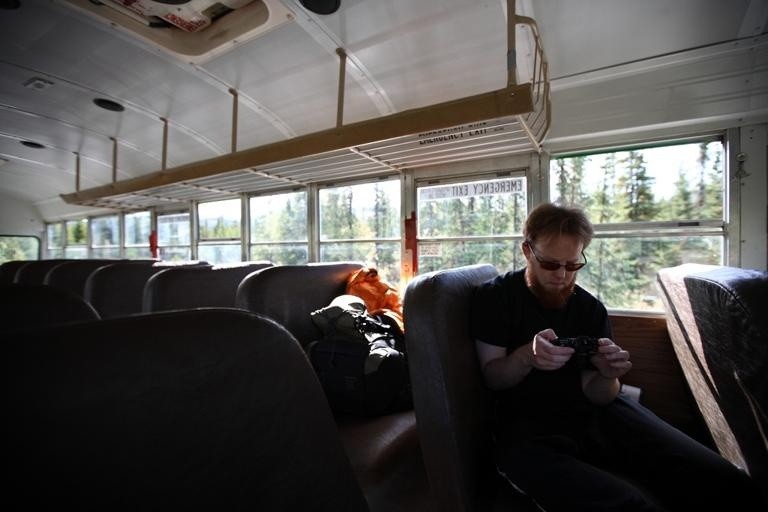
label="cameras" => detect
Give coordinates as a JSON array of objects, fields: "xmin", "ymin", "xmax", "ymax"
[{"xmin": 552, "ymin": 336, "xmax": 599, "ymax": 355}]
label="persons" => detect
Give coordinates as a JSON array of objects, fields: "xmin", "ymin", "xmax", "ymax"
[{"xmin": 467, "ymin": 202, "xmax": 767, "ymax": 512}]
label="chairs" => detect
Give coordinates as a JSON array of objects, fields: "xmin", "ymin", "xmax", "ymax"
[
  {"xmin": 402, "ymin": 263, "xmax": 643, "ymax": 512},
  {"xmin": 1, "ymin": 260, "xmax": 417, "ymax": 511},
  {"xmin": 655, "ymin": 262, "xmax": 767, "ymax": 484}
]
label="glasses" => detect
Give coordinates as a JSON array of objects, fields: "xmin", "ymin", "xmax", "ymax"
[{"xmin": 528, "ymin": 244, "xmax": 587, "ymax": 272}]
